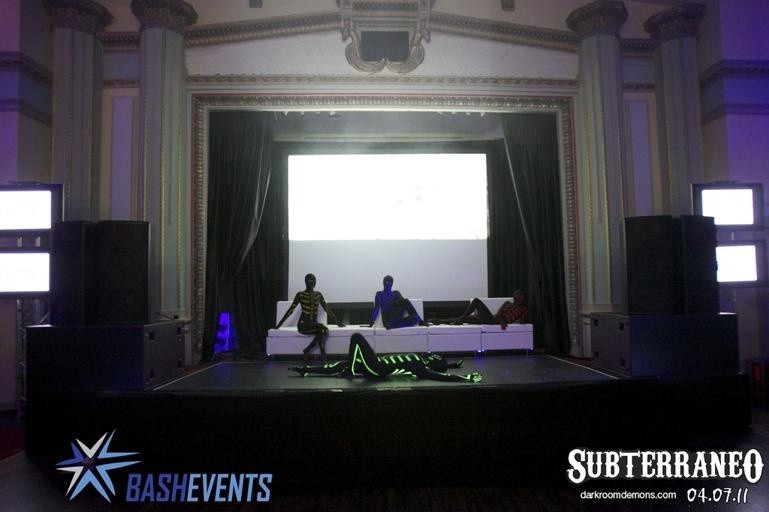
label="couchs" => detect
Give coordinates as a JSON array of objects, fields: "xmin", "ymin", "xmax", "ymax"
[{"xmin": 265, "ymin": 298, "xmax": 535, "ymax": 363}]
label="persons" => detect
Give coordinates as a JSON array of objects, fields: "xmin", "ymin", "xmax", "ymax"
[
  {"xmin": 427, "ymin": 286, "xmax": 531, "ymax": 330},
  {"xmin": 287, "ymin": 333, "xmax": 483, "ymax": 384},
  {"xmin": 274, "ymin": 273, "xmax": 345, "ymax": 365},
  {"xmin": 365, "ymin": 276, "xmax": 430, "ymax": 330}
]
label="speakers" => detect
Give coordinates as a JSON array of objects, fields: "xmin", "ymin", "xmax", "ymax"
[
  {"xmin": 50, "ymin": 219, "xmax": 96, "ymax": 327},
  {"xmin": 95, "ymin": 220, "xmax": 148, "ymax": 325},
  {"xmin": 672, "ymin": 215, "xmax": 719, "ymax": 314},
  {"xmin": 625, "ymin": 216, "xmax": 676, "ymax": 314}
]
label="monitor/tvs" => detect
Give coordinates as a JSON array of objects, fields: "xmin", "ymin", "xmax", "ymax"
[
  {"xmin": 0, "ymin": 183, "xmax": 63, "ymax": 237},
  {"xmin": 715, "ymin": 238, "xmax": 769, "ymax": 288},
  {"xmin": 0, "ymin": 247, "xmax": 50, "ymax": 299},
  {"xmin": 691, "ymin": 182, "xmax": 764, "ymax": 231}
]
